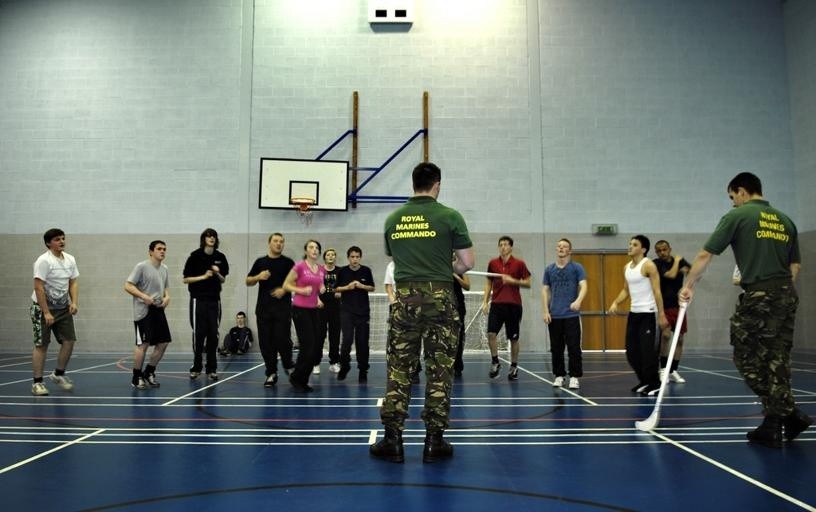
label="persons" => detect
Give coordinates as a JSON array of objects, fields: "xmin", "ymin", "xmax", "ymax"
[
  {"xmin": 650, "ymin": 240, "xmax": 690, "ymax": 383},
  {"xmin": 218, "ymin": 311, "xmax": 254, "ymax": 356},
  {"xmin": 29, "ymin": 229, "xmax": 80, "ymax": 395},
  {"xmin": 483, "ymin": 236, "xmax": 532, "ymax": 380},
  {"xmin": 182, "ymin": 229, "xmax": 229, "ymax": 381},
  {"xmin": 385, "ymin": 260, "xmax": 423, "ymax": 383},
  {"xmin": 542, "ymin": 238, "xmax": 588, "ymax": 389},
  {"xmin": 450, "ymin": 250, "xmax": 470, "ymax": 377},
  {"xmin": 677, "ymin": 171, "xmax": 814, "ymax": 445},
  {"xmin": 125, "ymin": 240, "xmax": 171, "ymax": 389},
  {"xmin": 370, "ymin": 162, "xmax": 477, "ymax": 465},
  {"xmin": 245, "ymin": 233, "xmax": 375, "ymax": 391},
  {"xmin": 607, "ymin": 235, "xmax": 670, "ymax": 396}
]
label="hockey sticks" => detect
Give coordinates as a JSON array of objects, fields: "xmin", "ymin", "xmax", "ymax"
[{"xmin": 632, "ymin": 304, "xmax": 687, "ymax": 432}]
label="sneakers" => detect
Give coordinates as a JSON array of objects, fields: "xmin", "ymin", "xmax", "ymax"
[
  {"xmin": 337, "ymin": 366, "xmax": 351, "ymax": 380},
  {"xmin": 142, "ymin": 372, "xmax": 161, "ymax": 388},
  {"xmin": 328, "ymin": 363, "xmax": 341, "ymax": 373},
  {"xmin": 47, "ymin": 369, "xmax": 74, "ymax": 391},
  {"xmin": 658, "ymin": 367, "xmax": 669, "ymax": 384},
  {"xmin": 669, "ymin": 370, "xmax": 687, "ymax": 384},
  {"xmin": 568, "ymin": 376, "xmax": 580, "ymax": 388},
  {"xmin": 488, "ymin": 362, "xmax": 503, "ymax": 380},
  {"xmin": 284, "ymin": 368, "xmax": 296, "ymax": 377},
  {"xmin": 507, "ymin": 365, "xmax": 519, "ymax": 381},
  {"xmin": 189, "ymin": 371, "xmax": 201, "ymax": 381},
  {"xmin": 631, "ymin": 382, "xmax": 649, "ymax": 393},
  {"xmin": 263, "ymin": 373, "xmax": 279, "ymax": 387},
  {"xmin": 130, "ymin": 375, "xmax": 148, "ymax": 390},
  {"xmin": 30, "ymin": 381, "xmax": 50, "ymax": 396},
  {"xmin": 358, "ymin": 370, "xmax": 368, "ymax": 383},
  {"xmin": 412, "ymin": 373, "xmax": 420, "ymax": 384},
  {"xmin": 552, "ymin": 376, "xmax": 566, "ymax": 387},
  {"xmin": 312, "ymin": 365, "xmax": 321, "ymax": 375},
  {"xmin": 640, "ymin": 381, "xmax": 661, "ymax": 396},
  {"xmin": 207, "ymin": 373, "xmax": 219, "ymax": 382},
  {"xmin": 454, "ymin": 368, "xmax": 462, "ymax": 380}
]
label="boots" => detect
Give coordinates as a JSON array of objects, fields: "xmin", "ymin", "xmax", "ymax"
[
  {"xmin": 782, "ymin": 408, "xmax": 813, "ymax": 442},
  {"xmin": 422, "ymin": 430, "xmax": 454, "ymax": 464},
  {"xmin": 368, "ymin": 426, "xmax": 405, "ymax": 465},
  {"xmin": 746, "ymin": 414, "xmax": 783, "ymax": 449}
]
